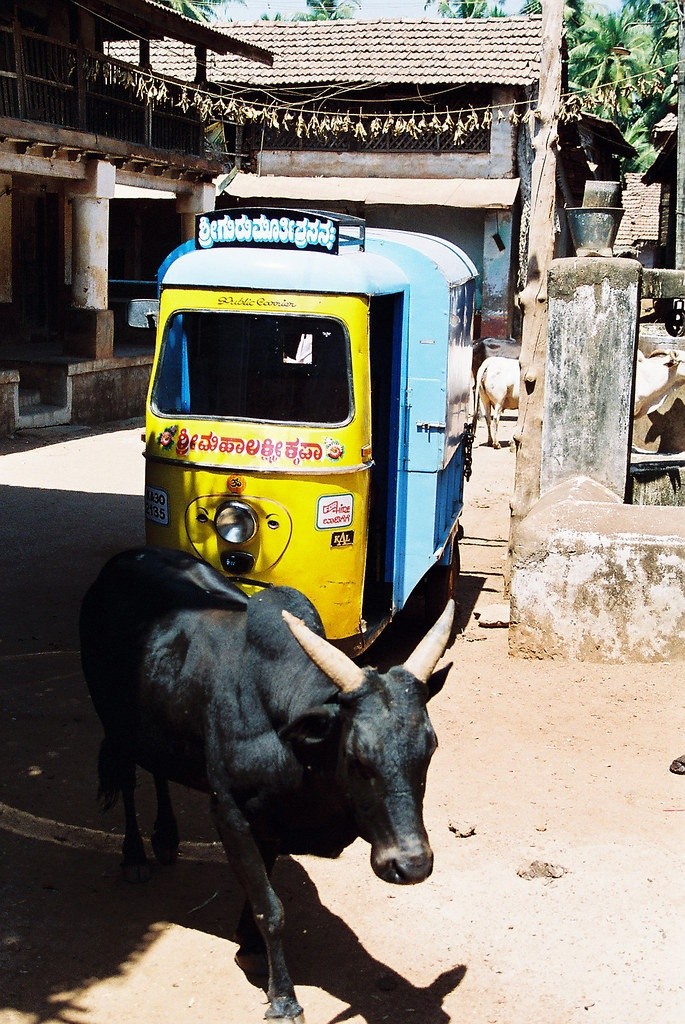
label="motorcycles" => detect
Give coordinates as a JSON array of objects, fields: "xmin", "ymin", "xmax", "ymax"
[{"xmin": 125, "ymin": 205, "xmax": 482, "ymax": 663}]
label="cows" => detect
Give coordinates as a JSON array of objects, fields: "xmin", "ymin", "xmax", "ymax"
[{"xmin": 77, "ymin": 544, "xmax": 461, "ymax": 1024}]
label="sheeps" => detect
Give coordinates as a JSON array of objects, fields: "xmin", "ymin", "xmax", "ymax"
[
  {"xmin": 472, "ymin": 335, "xmax": 521, "ymax": 450},
  {"xmin": 632, "ymin": 346, "xmax": 685, "ymax": 455}
]
